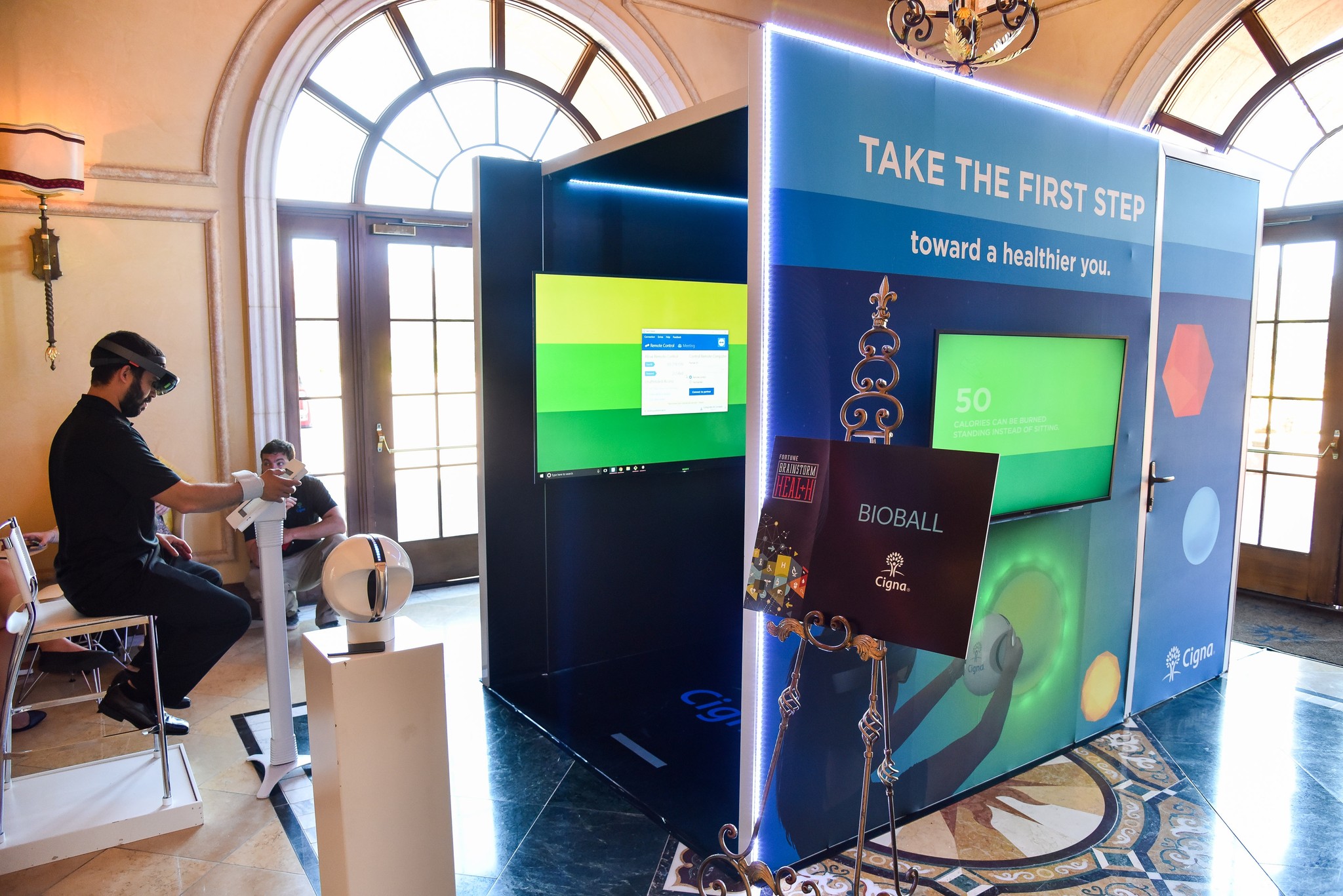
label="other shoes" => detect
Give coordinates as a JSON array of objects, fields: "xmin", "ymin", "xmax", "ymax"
[
  {"xmin": 286, "ymin": 612, "xmax": 300, "ymax": 629},
  {"xmin": 319, "ymin": 621, "xmax": 341, "ymax": 629}
]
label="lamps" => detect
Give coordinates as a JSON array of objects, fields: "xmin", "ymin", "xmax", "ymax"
[
  {"xmin": 0, "ymin": 123, "xmax": 87, "ymax": 370},
  {"xmin": 889, "ymin": 0, "xmax": 1039, "ymax": 77}
]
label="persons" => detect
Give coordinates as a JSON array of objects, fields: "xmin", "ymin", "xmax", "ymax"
[
  {"xmin": 776, "ymin": 624, "xmax": 1023, "ymax": 860},
  {"xmin": 48, "ymin": 330, "xmax": 302, "ymax": 736},
  {"xmin": 242, "ymin": 439, "xmax": 348, "ymax": 630}
]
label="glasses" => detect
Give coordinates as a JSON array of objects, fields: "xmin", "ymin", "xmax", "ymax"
[{"xmin": 152, "ymin": 371, "xmax": 177, "ymax": 395}]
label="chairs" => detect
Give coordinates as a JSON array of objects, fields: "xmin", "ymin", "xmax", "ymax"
[{"xmin": 0, "ymin": 509, "xmax": 185, "ymax": 805}]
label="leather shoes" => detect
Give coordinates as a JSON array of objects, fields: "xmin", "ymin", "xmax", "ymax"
[
  {"xmin": 38, "ymin": 650, "xmax": 113, "ymax": 674},
  {"xmin": 113, "ymin": 669, "xmax": 191, "ymax": 710},
  {"xmin": 11, "ymin": 710, "xmax": 47, "ymax": 732},
  {"xmin": 101, "ymin": 681, "xmax": 189, "ymax": 735}
]
